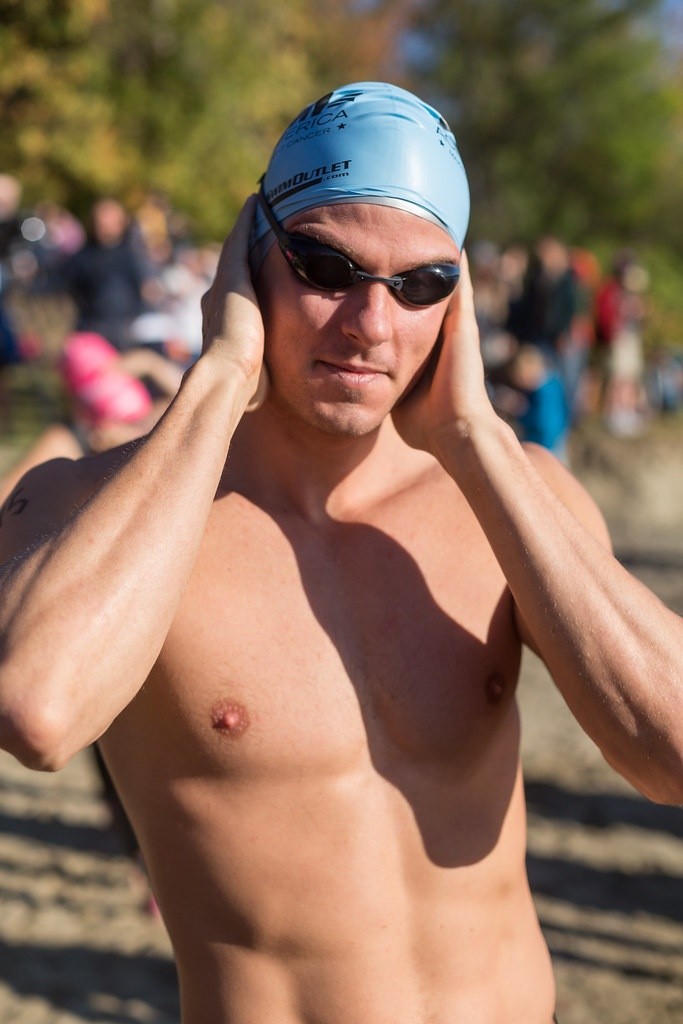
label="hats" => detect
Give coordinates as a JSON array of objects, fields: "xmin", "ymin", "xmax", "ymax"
[
  {"xmin": 252, "ymin": 80, "xmax": 469, "ymax": 248},
  {"xmin": 62, "ymin": 332, "xmax": 152, "ymax": 427}
]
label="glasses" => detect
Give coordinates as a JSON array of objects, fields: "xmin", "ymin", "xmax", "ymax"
[{"xmin": 256, "ymin": 173, "xmax": 460, "ymax": 306}]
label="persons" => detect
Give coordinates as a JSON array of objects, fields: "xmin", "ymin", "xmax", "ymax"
[{"xmin": 0, "ymin": 83, "xmax": 683, "ymax": 1024}]
